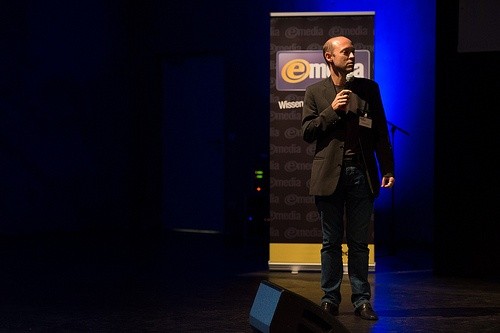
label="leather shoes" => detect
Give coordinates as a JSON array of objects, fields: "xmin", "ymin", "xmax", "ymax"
[
  {"xmin": 319, "ymin": 300, "xmax": 339, "ymax": 317},
  {"xmin": 354, "ymin": 301, "xmax": 377, "ymax": 321}
]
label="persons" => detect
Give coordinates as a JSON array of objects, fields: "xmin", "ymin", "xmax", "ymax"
[{"xmin": 301, "ymin": 35, "xmax": 397, "ymax": 320}]
